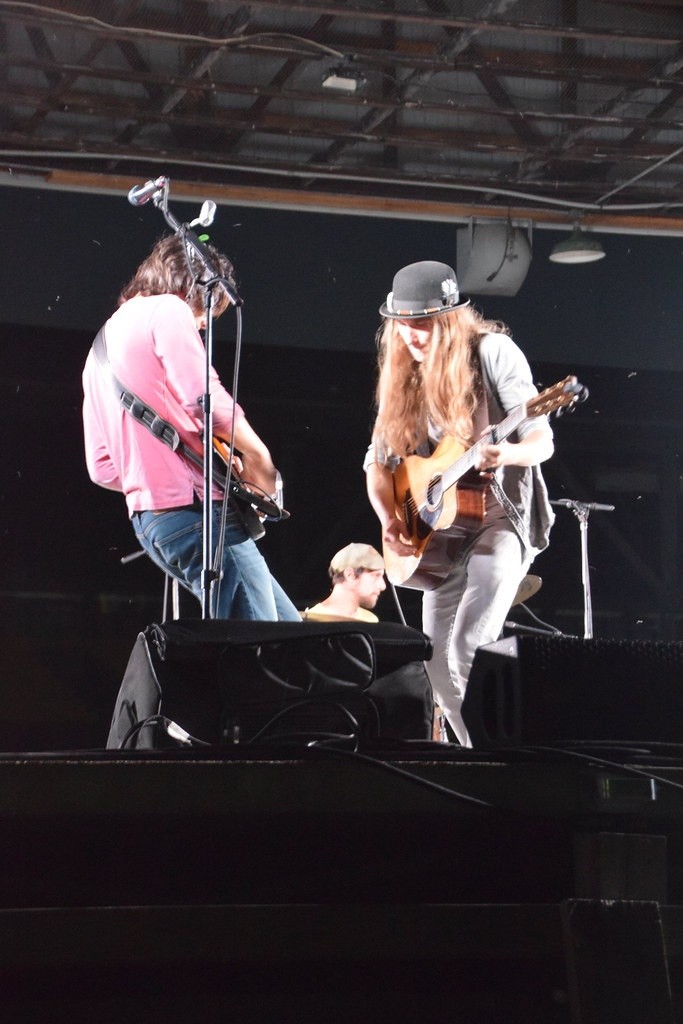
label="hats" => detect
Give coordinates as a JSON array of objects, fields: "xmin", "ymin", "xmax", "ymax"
[
  {"xmin": 379, "ymin": 260, "xmax": 472, "ymax": 320},
  {"xmin": 330, "ymin": 542, "xmax": 385, "ymax": 572}
]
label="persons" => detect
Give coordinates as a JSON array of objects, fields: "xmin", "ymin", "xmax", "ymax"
[
  {"xmin": 297, "ymin": 543, "xmax": 385, "ymax": 622},
  {"xmin": 79, "ymin": 237, "xmax": 305, "ymax": 623},
  {"xmin": 364, "ymin": 260, "xmax": 556, "ymax": 750}
]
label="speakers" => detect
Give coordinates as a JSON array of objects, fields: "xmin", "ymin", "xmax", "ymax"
[
  {"xmin": 459, "ymin": 633, "xmax": 683, "ymax": 750},
  {"xmin": 98, "ymin": 620, "xmax": 437, "ymax": 748}
]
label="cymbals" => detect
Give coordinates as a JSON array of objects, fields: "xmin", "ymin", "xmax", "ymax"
[{"xmin": 511, "ymin": 574, "xmax": 542, "ymax": 608}]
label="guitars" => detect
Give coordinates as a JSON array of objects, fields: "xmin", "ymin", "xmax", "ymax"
[
  {"xmin": 203, "ymin": 430, "xmax": 268, "ymax": 541},
  {"xmin": 381, "ymin": 374, "xmax": 588, "ymax": 590}
]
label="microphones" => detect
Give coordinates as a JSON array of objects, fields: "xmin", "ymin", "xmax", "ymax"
[{"xmin": 128, "ymin": 176, "xmax": 169, "ymax": 206}]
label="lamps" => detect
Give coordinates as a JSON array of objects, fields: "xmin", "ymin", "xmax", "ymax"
[
  {"xmin": 321, "ymin": 63, "xmax": 368, "ymax": 93},
  {"xmin": 549, "ymin": 212, "xmax": 607, "ymax": 267}
]
